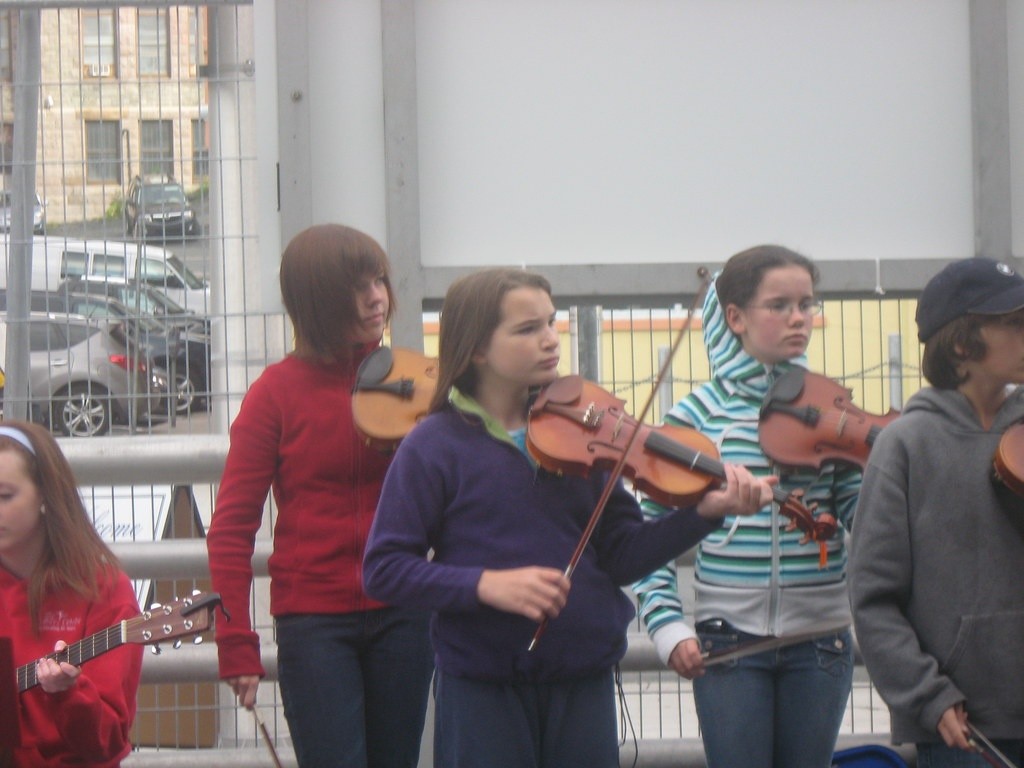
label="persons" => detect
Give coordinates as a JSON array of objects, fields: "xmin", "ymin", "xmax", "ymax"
[
  {"xmin": 632, "ymin": 244, "xmax": 863, "ymax": 768},
  {"xmin": 0, "ymin": 417, "xmax": 145, "ymax": 768},
  {"xmin": 359, "ymin": 270, "xmax": 778, "ymax": 768},
  {"xmin": 203, "ymin": 222, "xmax": 434, "ymax": 768},
  {"xmin": 849, "ymin": 257, "xmax": 1023, "ymax": 768}
]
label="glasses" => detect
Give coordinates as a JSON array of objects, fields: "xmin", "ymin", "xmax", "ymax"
[{"xmin": 738, "ymin": 298, "xmax": 823, "ymax": 317}]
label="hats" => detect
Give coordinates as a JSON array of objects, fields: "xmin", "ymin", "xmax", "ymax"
[{"xmin": 915, "ymin": 258, "xmax": 1024, "ymax": 344}]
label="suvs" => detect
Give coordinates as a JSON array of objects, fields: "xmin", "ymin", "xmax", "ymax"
[
  {"xmin": 126, "ymin": 174, "xmax": 198, "ymax": 245},
  {"xmin": 0, "ymin": 234, "xmax": 211, "ymax": 440}
]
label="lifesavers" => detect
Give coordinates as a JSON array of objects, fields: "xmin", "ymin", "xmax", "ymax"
[{"xmin": 15, "ymin": 587, "xmax": 217, "ymax": 695}]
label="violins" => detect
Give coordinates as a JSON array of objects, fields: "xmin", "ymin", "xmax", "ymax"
[
  {"xmin": 993, "ymin": 417, "xmax": 1024, "ymax": 496},
  {"xmin": 350, "ymin": 346, "xmax": 441, "ymax": 451},
  {"xmin": 757, "ymin": 369, "xmax": 903, "ymax": 477},
  {"xmin": 525, "ymin": 375, "xmax": 838, "ymax": 546}
]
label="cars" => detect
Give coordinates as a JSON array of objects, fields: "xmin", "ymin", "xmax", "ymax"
[{"xmin": 0, "ymin": 189, "xmax": 49, "ymax": 235}]
